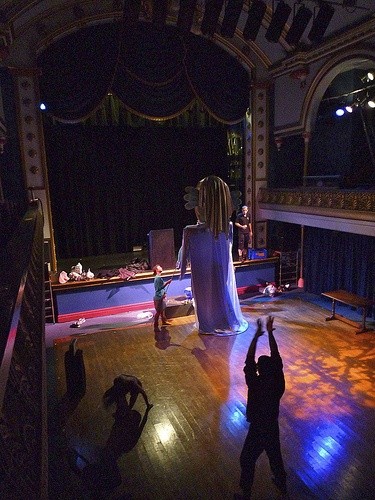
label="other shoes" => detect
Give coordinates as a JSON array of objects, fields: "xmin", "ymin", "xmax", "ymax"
[
  {"xmin": 272, "ymin": 473, "xmax": 287, "ymax": 487},
  {"xmin": 233, "ymin": 492, "xmax": 252, "ymax": 500}
]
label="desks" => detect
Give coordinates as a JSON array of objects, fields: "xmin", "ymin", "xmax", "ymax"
[{"xmin": 321, "ymin": 289, "xmax": 375, "ymax": 335}]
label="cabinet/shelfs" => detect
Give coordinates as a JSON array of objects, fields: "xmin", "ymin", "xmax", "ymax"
[{"xmin": 272, "ymin": 248, "xmax": 301, "ymax": 288}]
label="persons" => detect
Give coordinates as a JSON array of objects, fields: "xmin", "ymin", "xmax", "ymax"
[
  {"xmin": 103, "ymin": 374, "xmax": 154, "ymax": 422},
  {"xmin": 243, "ymin": 282, "xmax": 284, "ymax": 297},
  {"xmin": 233, "ymin": 315, "xmax": 288, "ymax": 500},
  {"xmin": 152, "ymin": 264, "xmax": 172, "ymax": 332},
  {"xmin": 235, "ymin": 206, "xmax": 253, "ymax": 263},
  {"xmin": 176, "ymin": 176, "xmax": 249, "ymax": 336}
]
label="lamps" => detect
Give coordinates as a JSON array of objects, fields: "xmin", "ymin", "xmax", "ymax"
[
  {"xmin": 220, "ymin": 0, "xmax": 245, "ymax": 39},
  {"xmin": 265, "ymin": 1, "xmax": 292, "ymax": 43},
  {"xmin": 335, "ymin": 99, "xmax": 347, "ymax": 116},
  {"xmin": 360, "ymin": 74, "xmax": 370, "ymax": 83},
  {"xmin": 132, "ymin": 245, "xmax": 143, "ymax": 251},
  {"xmin": 307, "ymin": 2, "xmax": 336, "ymax": 43},
  {"xmin": 367, "ymin": 69, "xmax": 375, "ymax": 80},
  {"xmin": 368, "ymin": 94, "xmax": 375, "ymax": 108},
  {"xmin": 200, "ymin": 0, "xmax": 225, "ymax": 36},
  {"xmin": 345, "ymin": 96, "xmax": 361, "ymax": 113},
  {"xmin": 120, "ymin": 0, "xmax": 146, "ymax": 27},
  {"xmin": 177, "ymin": 0, "xmax": 198, "ymax": 32},
  {"xmin": 359, "ymin": 91, "xmax": 373, "ymax": 110},
  {"xmin": 285, "ymin": 2, "xmax": 313, "ymax": 44},
  {"xmin": 242, "ymin": 0, "xmax": 267, "ymax": 41},
  {"xmin": 151, "ymin": 0, "xmax": 172, "ymax": 31}
]
